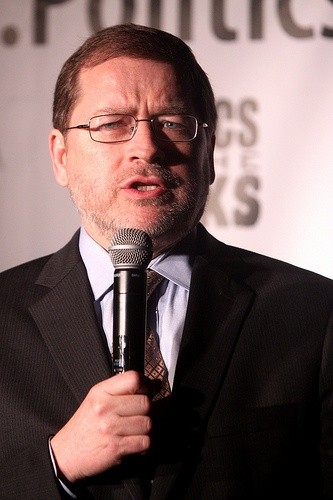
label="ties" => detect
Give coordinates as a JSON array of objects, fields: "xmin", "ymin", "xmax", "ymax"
[{"xmin": 138, "ymin": 268, "xmax": 170, "ymax": 406}]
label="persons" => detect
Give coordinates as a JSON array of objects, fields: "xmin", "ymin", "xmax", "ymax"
[{"xmin": 0, "ymin": 21, "xmax": 333, "ymax": 500}]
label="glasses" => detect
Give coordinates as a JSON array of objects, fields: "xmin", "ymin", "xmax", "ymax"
[{"xmin": 61, "ymin": 114, "xmax": 211, "ymax": 143}]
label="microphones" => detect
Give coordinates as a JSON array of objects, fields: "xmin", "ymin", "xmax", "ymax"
[{"xmin": 107, "ymin": 228, "xmax": 155, "ymax": 379}]
